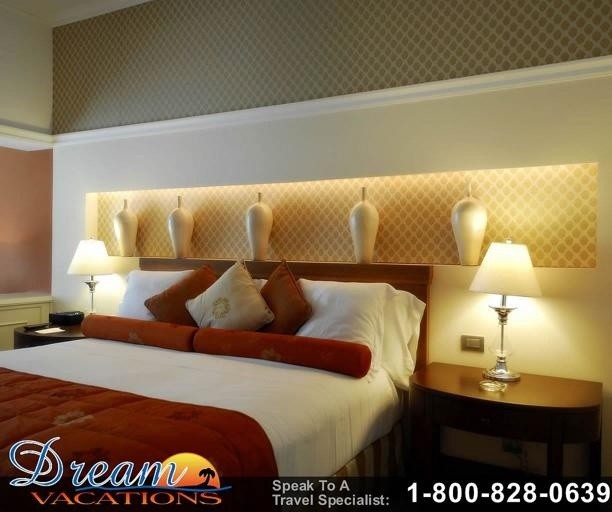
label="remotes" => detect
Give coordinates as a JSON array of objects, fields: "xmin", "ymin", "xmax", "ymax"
[{"xmin": 24, "ymin": 322, "xmax": 50, "ymax": 328}]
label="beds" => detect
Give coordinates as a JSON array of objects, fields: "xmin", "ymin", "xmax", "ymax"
[{"xmin": 0, "ymin": 258, "xmax": 434, "ymax": 480}]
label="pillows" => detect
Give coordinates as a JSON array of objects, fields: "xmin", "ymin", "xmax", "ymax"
[
  {"xmin": 295, "ymin": 278, "xmax": 387, "ymax": 352},
  {"xmin": 117, "ymin": 270, "xmax": 192, "ymax": 322},
  {"xmin": 185, "ymin": 259, "xmax": 274, "ymax": 330},
  {"xmin": 143, "ymin": 263, "xmax": 217, "ymax": 329},
  {"xmin": 261, "ymin": 259, "xmax": 312, "ymax": 336}
]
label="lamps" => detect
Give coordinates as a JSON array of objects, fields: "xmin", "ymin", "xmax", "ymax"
[
  {"xmin": 66, "ymin": 239, "xmax": 116, "ymax": 314},
  {"xmin": 468, "ymin": 243, "xmax": 541, "ymax": 382}
]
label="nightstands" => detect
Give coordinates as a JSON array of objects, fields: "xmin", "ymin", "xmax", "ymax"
[
  {"xmin": 13, "ymin": 321, "xmax": 85, "ymax": 350},
  {"xmin": 407, "ymin": 361, "xmax": 604, "ymax": 488}
]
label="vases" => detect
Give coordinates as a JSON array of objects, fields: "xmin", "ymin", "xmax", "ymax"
[
  {"xmin": 112, "ymin": 199, "xmax": 138, "ymax": 258},
  {"xmin": 245, "ymin": 192, "xmax": 273, "ymax": 259},
  {"xmin": 451, "ymin": 182, "xmax": 487, "ymax": 266},
  {"xmin": 348, "ymin": 187, "xmax": 380, "ymax": 263},
  {"xmin": 168, "ymin": 196, "xmax": 194, "ymax": 258}
]
